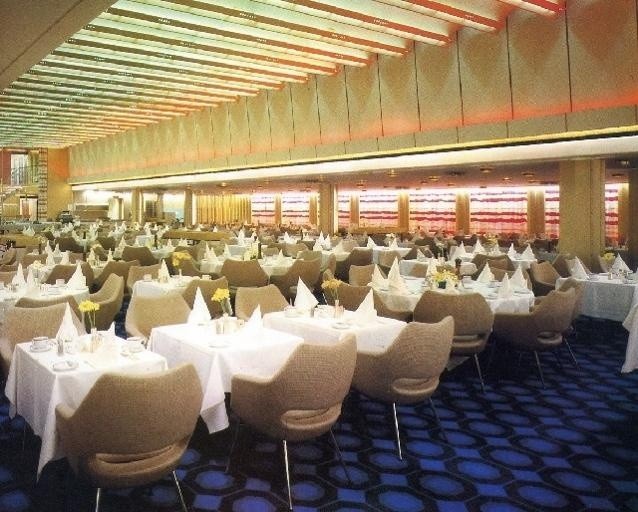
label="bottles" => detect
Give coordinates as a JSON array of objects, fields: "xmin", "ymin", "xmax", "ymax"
[
  {"xmin": 57, "ymin": 336, "xmax": 64, "ymax": 357},
  {"xmin": 90, "ymin": 335, "xmax": 95, "ymax": 353}
]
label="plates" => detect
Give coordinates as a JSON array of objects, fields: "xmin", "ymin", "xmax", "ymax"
[
  {"xmin": 53, "ymin": 362, "xmax": 78, "ymax": 370},
  {"xmin": 30, "ymin": 345, "xmax": 52, "ymax": 351}
]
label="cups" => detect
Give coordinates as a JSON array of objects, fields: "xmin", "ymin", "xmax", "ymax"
[
  {"xmin": 0, "ymin": 276, "xmax": 86, "ymax": 298},
  {"xmin": 32, "ymin": 337, "xmax": 48, "ymax": 348},
  {"xmin": 589, "ymin": 267, "xmax": 633, "ymax": 284},
  {"xmin": 123, "ymin": 273, "xmax": 530, "ymax": 353}
]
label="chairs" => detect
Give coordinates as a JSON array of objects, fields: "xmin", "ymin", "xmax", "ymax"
[
  {"xmin": 483, "ymin": 286, "xmax": 584, "ymax": 388},
  {"xmin": 2, "ymin": 237, "xmax": 596, "ymax": 483},
  {"xmin": 408, "ymin": 289, "xmax": 496, "ymax": 399},
  {"xmin": 227, "ymin": 330, "xmax": 358, "ymax": 510},
  {"xmin": 51, "ymin": 360, "xmax": 207, "ymax": 512},
  {"xmin": 355, "ymin": 315, "xmax": 461, "ymax": 460}
]
